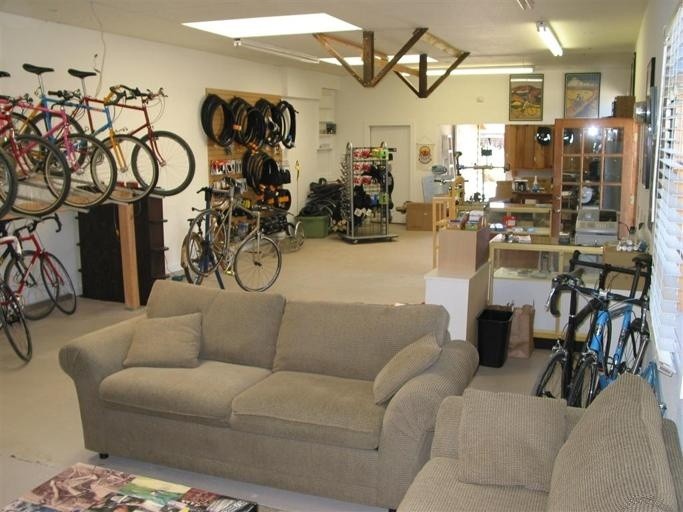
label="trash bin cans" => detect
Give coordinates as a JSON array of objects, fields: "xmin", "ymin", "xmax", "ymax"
[{"xmin": 476, "ymin": 309, "xmax": 514, "ymax": 368}]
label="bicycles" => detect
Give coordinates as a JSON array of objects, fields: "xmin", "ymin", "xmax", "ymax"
[
  {"xmin": 181, "ymin": 174, "xmax": 282, "ymax": 292},
  {"xmin": 530, "ymin": 250, "xmax": 652, "ymax": 408}
]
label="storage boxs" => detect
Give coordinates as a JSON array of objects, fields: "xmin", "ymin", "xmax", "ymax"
[
  {"xmin": 436, "ymin": 227, "xmax": 488, "ymax": 275},
  {"xmin": 294, "ymin": 215, "xmax": 329, "ymax": 239}
]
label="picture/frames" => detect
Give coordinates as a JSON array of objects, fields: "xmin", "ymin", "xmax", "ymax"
[
  {"xmin": 508, "ymin": 73, "xmax": 545, "ymax": 122},
  {"xmin": 563, "ymin": 72, "xmax": 601, "ymax": 120}
]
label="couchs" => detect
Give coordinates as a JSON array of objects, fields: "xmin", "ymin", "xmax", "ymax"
[
  {"xmin": 398, "ymin": 375, "xmax": 683, "ymax": 512},
  {"xmin": 56, "ymin": 277, "xmax": 481, "ymax": 512}
]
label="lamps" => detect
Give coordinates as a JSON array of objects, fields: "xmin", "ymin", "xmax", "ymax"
[
  {"xmin": 535, "ymin": 18, "xmax": 564, "ymax": 58},
  {"xmin": 515, "ymin": 0, "xmax": 533, "ymax": 14},
  {"xmin": 233, "ymin": 37, "xmax": 320, "ymax": 65}
]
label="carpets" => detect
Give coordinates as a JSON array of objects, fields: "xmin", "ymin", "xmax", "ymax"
[{"xmin": 256, "ymin": 505, "xmax": 288, "ymax": 512}]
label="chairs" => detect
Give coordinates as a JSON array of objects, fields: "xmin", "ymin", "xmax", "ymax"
[{"xmin": 432, "ymin": 196, "xmax": 454, "ymax": 270}]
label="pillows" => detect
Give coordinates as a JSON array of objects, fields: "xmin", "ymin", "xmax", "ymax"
[
  {"xmin": 456, "ymin": 386, "xmax": 569, "ymax": 493},
  {"xmin": 372, "ymin": 331, "xmax": 443, "ymax": 408},
  {"xmin": 122, "ymin": 312, "xmax": 204, "ymax": 368}
]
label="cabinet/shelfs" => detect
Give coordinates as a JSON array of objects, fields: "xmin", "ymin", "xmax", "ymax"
[
  {"xmin": 503, "ymin": 124, "xmax": 555, "ymax": 172},
  {"xmin": 424, "ymin": 258, "xmax": 490, "ymax": 346},
  {"xmin": 338, "ymin": 141, "xmax": 400, "ymax": 244},
  {"xmin": 74, "ymin": 183, "xmax": 169, "ymax": 307},
  {"xmin": 486, "ymin": 200, "xmax": 552, "ymax": 236},
  {"xmin": 487, "ymin": 233, "xmax": 605, "ymax": 343},
  {"xmin": 406, "ymin": 200, "xmax": 443, "ymax": 230}
]
